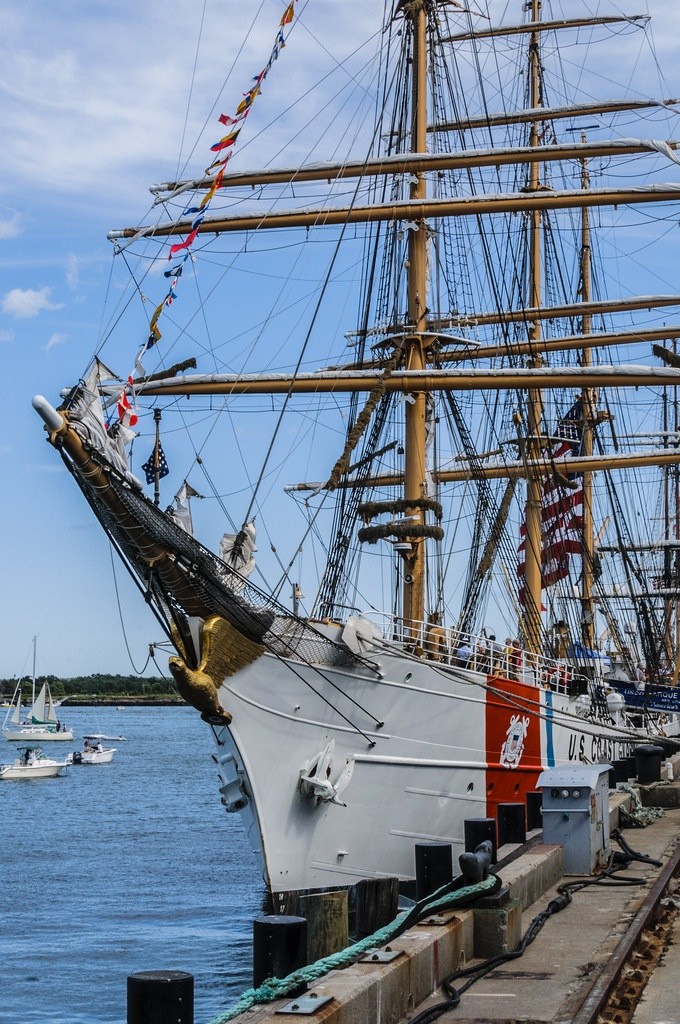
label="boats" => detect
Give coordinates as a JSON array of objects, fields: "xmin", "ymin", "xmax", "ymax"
[
  {"xmin": 0, "ymin": 701, "xmax": 24, "ymax": 709},
  {"xmin": 64, "ymin": 746, "xmax": 116, "ymax": 764},
  {"xmin": 0, "ymin": 746, "xmax": 67, "ymax": 781},
  {"xmin": 84, "ymin": 733, "xmax": 126, "ymax": 742}
]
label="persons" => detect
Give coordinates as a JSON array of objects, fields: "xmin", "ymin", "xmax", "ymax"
[
  {"xmin": 425, "ymin": 612, "xmax": 439, "ymax": 640},
  {"xmin": 84, "ymin": 740, "xmax": 103, "ymax": 754},
  {"xmin": 457, "ymin": 635, "xmax": 522, "ymax": 681},
  {"xmin": 23, "ymin": 719, "xmax": 29, "ymax": 725},
  {"xmin": 541, "ymin": 665, "xmax": 572, "ymax": 694},
  {"xmin": 634, "ymin": 662, "xmax": 670, "ymax": 691},
  {"xmin": 56, "ymin": 720, "xmax": 67, "ymax": 732}
]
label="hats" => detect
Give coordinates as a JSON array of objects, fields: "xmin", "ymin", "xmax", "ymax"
[
  {"xmin": 504, "ymin": 638, "xmax": 511, "ymax": 641},
  {"xmin": 489, "ymin": 635, "xmax": 496, "ymax": 640},
  {"xmin": 512, "ymin": 639, "xmax": 519, "ymax": 643}
]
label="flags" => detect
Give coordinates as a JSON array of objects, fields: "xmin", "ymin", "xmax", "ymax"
[
  {"xmin": 108, "ymin": 0, "xmax": 295, "ymax": 445},
  {"xmin": 141, "ymin": 439, "xmax": 169, "ymax": 485}
]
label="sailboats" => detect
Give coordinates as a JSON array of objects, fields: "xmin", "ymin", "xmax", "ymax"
[
  {"xmin": 30, "ymin": 0, "xmax": 680, "ymax": 923},
  {"xmin": 0, "ymin": 635, "xmax": 74, "ymax": 742}
]
